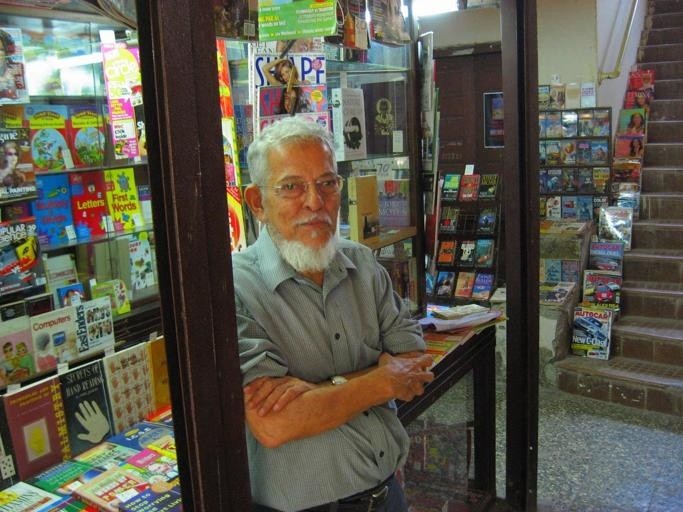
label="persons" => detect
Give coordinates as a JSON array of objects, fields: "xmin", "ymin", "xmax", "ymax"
[{"xmin": 232, "ymin": 117, "xmax": 434, "ymax": 511}]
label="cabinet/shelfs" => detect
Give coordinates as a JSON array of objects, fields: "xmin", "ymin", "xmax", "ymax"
[
  {"xmin": 426, "ymin": 167, "xmax": 505, "ymax": 312},
  {"xmin": 2, "ymin": 5, "xmax": 426, "ymax": 490}
]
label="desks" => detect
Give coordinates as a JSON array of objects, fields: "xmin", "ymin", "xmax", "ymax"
[{"xmin": 1, "ymin": 316, "xmax": 501, "ymax": 510}]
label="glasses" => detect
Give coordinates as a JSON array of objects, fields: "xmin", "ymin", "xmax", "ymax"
[{"xmin": 257, "ymin": 174, "xmax": 345, "ymax": 199}]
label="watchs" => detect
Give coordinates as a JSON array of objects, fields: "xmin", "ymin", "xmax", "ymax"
[{"xmin": 331, "ymin": 375, "xmax": 348, "ymax": 385}]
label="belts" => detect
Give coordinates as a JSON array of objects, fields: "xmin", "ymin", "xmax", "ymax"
[{"xmin": 306, "ymin": 485, "xmax": 390, "ymax": 512}]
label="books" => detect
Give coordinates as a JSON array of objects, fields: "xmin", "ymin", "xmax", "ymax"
[
  {"xmin": 348, "ymin": 175, "xmax": 418, "ymax": 311},
  {"xmin": 539, "ymin": 70, "xmax": 654, "ymax": 359},
  {"xmin": 0, "ymin": 26, "xmax": 181, "ymax": 512},
  {"xmin": 248, "ymin": 37, "xmax": 409, "ymax": 162},
  {"xmin": 435, "ymin": 174, "xmax": 507, "ymax": 304}
]
